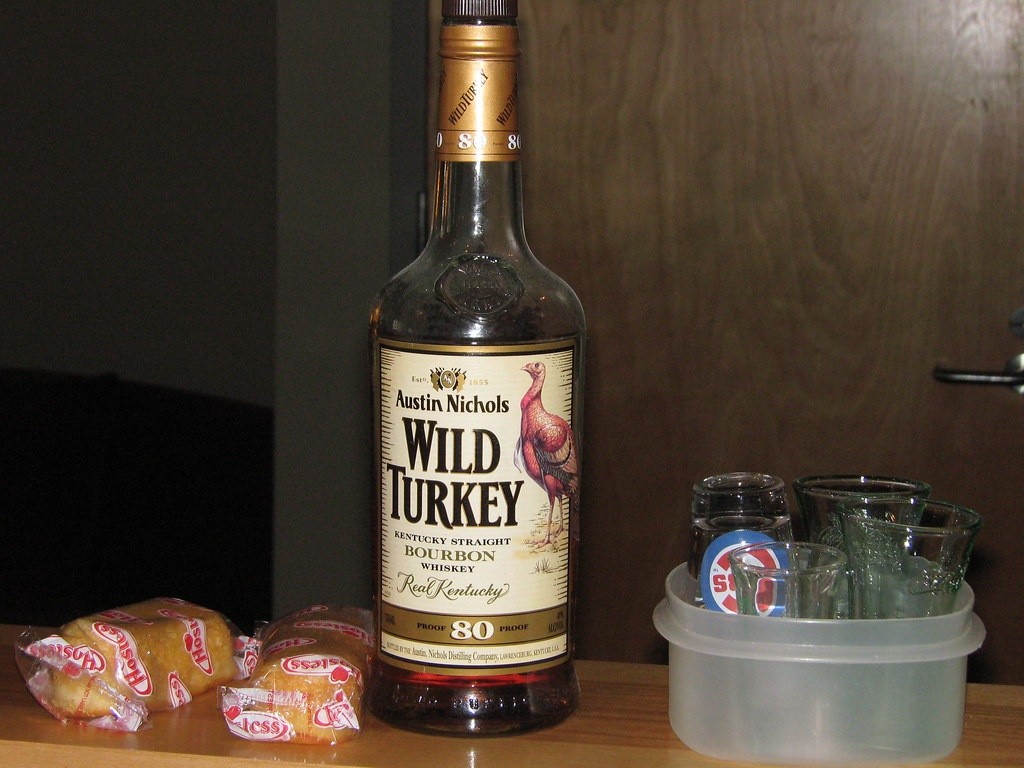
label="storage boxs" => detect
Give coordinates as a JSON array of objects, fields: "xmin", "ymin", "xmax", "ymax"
[{"xmin": 652, "ymin": 559, "xmax": 987, "ymax": 763}]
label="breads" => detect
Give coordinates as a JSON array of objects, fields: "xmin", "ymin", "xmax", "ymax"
[
  {"xmin": 240, "ymin": 603, "xmax": 376, "ymax": 746},
  {"xmin": 45, "ymin": 597, "xmax": 238, "ymax": 718}
]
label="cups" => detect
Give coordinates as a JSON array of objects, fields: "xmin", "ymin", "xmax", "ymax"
[
  {"xmin": 730, "ymin": 541, "xmax": 847, "ymax": 621},
  {"xmin": 792, "ymin": 474, "xmax": 932, "ymax": 557},
  {"xmin": 839, "ymin": 500, "xmax": 984, "ymax": 620},
  {"xmin": 687, "ymin": 469, "xmax": 793, "ymax": 620}
]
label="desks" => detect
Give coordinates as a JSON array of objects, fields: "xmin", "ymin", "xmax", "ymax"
[{"xmin": 0, "ymin": 622, "xmax": 1023, "ymax": 768}]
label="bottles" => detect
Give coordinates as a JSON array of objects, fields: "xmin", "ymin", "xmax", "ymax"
[{"xmin": 368, "ymin": 0, "xmax": 583, "ymax": 737}]
